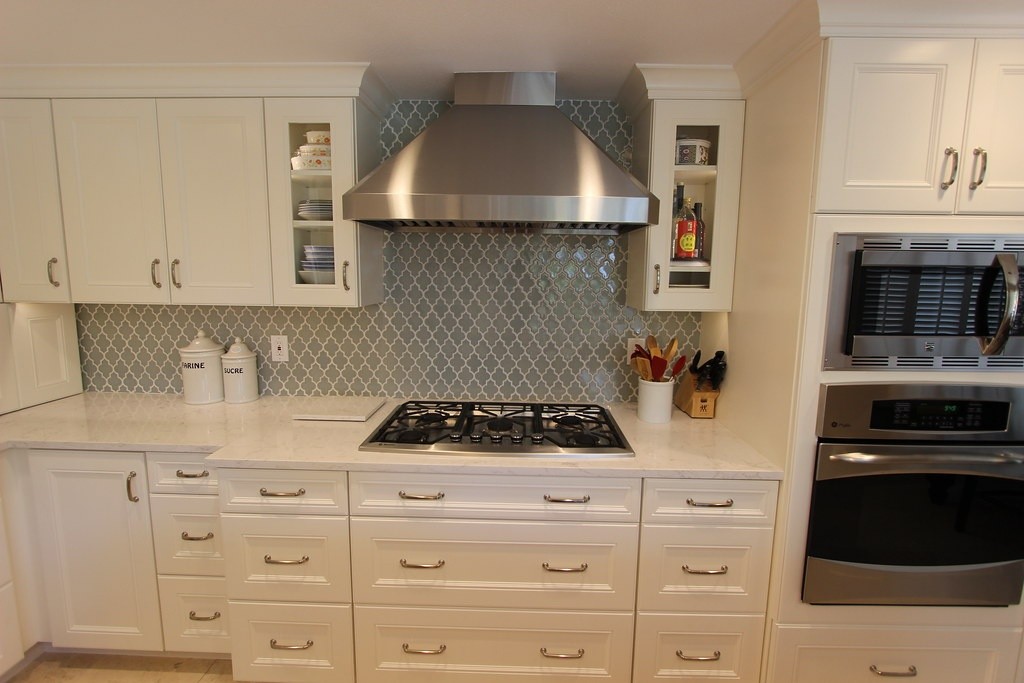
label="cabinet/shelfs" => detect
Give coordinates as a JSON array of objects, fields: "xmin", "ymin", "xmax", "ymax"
[
  {"xmin": 0, "ymin": 99, "xmax": 385, "ymax": 416},
  {"xmin": 627, "ymin": 101, "xmax": 744, "ymax": 314},
  {"xmin": 0, "ymin": 450, "xmax": 1024, "ymax": 683},
  {"xmin": 741, "ymin": 37, "xmax": 1024, "ymax": 218}
]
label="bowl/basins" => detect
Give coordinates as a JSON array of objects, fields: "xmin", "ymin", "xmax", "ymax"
[
  {"xmin": 299, "ymin": 245, "xmax": 334, "ymax": 285},
  {"xmin": 290, "ymin": 130, "xmax": 331, "ymax": 169},
  {"xmin": 675, "ymin": 138, "xmax": 712, "ymax": 166}
]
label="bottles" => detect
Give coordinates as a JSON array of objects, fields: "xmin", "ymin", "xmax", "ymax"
[
  {"xmin": 180, "ymin": 329, "xmax": 225, "ymax": 404},
  {"xmin": 221, "ymin": 337, "xmax": 259, "ymax": 403},
  {"xmin": 672, "ymin": 179, "xmax": 706, "ymax": 262}
]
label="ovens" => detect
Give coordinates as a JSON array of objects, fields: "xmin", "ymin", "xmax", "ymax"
[{"xmin": 802, "ymin": 380, "xmax": 1024, "ymax": 605}]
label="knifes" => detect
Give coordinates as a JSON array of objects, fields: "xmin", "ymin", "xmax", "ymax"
[{"xmin": 689, "ymin": 347, "xmax": 726, "ymax": 390}]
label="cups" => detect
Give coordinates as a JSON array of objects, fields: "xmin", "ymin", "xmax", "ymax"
[{"xmin": 637, "ymin": 377, "xmax": 674, "ymax": 425}]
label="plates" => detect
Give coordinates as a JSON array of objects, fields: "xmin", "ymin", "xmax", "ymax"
[
  {"xmin": 298, "ymin": 199, "xmax": 334, "ymax": 220},
  {"xmin": 668, "ymin": 284, "xmax": 709, "ymax": 287}
]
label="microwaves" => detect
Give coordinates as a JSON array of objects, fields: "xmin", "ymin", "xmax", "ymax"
[{"xmin": 821, "ymin": 233, "xmax": 1024, "ymax": 371}]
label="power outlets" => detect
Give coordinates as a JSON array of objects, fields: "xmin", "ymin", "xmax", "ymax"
[
  {"xmin": 271, "ymin": 335, "xmax": 289, "ymax": 361},
  {"xmin": 627, "ymin": 338, "xmax": 645, "ymax": 366}
]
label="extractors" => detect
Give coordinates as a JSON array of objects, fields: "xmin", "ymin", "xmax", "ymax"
[{"xmin": 342, "ymin": 71, "xmax": 660, "ymax": 236}]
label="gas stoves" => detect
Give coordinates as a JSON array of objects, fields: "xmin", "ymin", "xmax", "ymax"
[{"xmin": 357, "ymin": 400, "xmax": 636, "ymax": 457}]
